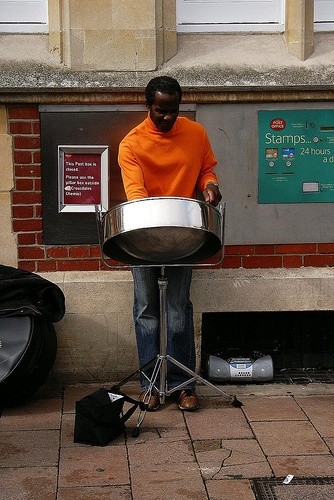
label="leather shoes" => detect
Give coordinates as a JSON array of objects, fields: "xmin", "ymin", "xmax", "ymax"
[
  {"xmin": 139, "ymin": 389, "xmax": 161, "ymax": 410},
  {"xmin": 178, "ymin": 389, "xmax": 199, "ymax": 411}
]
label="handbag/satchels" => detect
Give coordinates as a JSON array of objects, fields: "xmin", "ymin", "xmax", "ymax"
[{"xmin": 74, "ymin": 388, "xmax": 139, "ymax": 447}]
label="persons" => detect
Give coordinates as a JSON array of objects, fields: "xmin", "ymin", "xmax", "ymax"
[{"xmin": 117, "ymin": 75, "xmax": 222, "ymax": 411}]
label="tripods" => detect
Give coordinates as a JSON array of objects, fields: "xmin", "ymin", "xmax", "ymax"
[{"xmin": 109, "ymin": 265, "xmax": 242, "ymax": 437}]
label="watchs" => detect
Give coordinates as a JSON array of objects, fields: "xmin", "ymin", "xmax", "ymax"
[{"xmin": 207, "ymin": 182, "xmax": 220, "ymax": 188}]
